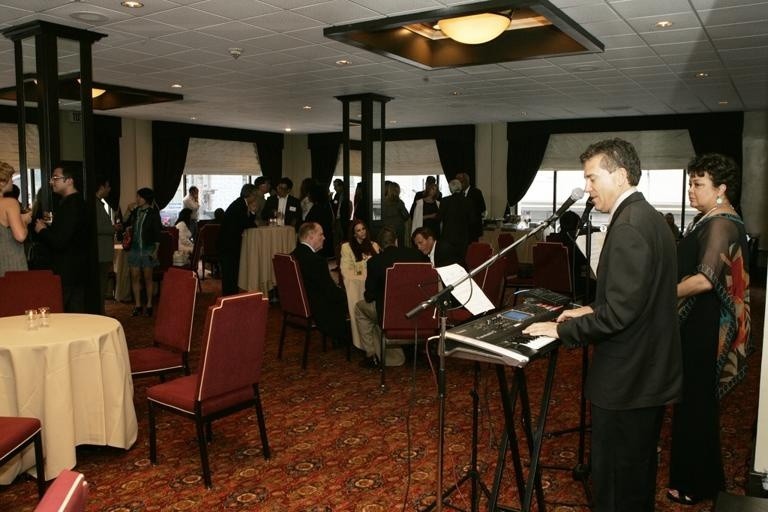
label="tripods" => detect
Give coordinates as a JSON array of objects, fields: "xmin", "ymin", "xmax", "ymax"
[
  {"xmin": 427, "ymin": 364, "xmax": 493, "ymax": 509},
  {"xmin": 526, "ymin": 220, "xmax": 597, "ymax": 511}
]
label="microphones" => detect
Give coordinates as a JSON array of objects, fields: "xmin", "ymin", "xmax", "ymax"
[
  {"xmin": 574, "ymin": 197, "xmax": 594, "ymax": 239},
  {"xmin": 552, "ymin": 188, "xmax": 584, "ymax": 221}
]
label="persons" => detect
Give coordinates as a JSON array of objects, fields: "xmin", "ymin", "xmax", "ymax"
[
  {"xmin": 411, "ymin": 228, "xmax": 446, "ymax": 269},
  {"xmin": 254, "ymin": 176, "xmax": 270, "ymax": 228},
  {"xmin": 457, "ymin": 172, "xmax": 486, "ymax": 242},
  {"xmin": 174, "ymin": 208, "xmax": 194, "ymax": 253},
  {"xmin": 352, "ymin": 182, "xmax": 365, "ymax": 220},
  {"xmin": 411, "ymin": 176, "xmax": 442, "ymax": 218},
  {"xmin": 262, "ymin": 176, "xmax": 301, "ymax": 225},
  {"xmin": 385, "ymin": 182, "xmax": 411, "ymax": 246},
  {"xmin": 332, "ymin": 179, "xmax": 350, "ymax": 239},
  {"xmin": 546, "ymin": 211, "xmax": 596, "ymax": 303},
  {"xmin": 34, "ymin": 165, "xmax": 100, "ymax": 314},
  {"xmin": 290, "ymin": 221, "xmax": 347, "ymax": 337},
  {"xmin": 216, "ymin": 184, "xmax": 257, "ymax": 295},
  {"xmin": 0, "ymin": 161, "xmax": 32, "ymax": 277},
  {"xmin": 341, "ymin": 221, "xmax": 381, "ymax": 287},
  {"xmin": 411, "ymin": 183, "xmax": 440, "ymax": 234},
  {"xmin": 303, "ymin": 179, "xmax": 334, "ymax": 257},
  {"xmin": 124, "ymin": 187, "xmax": 161, "ymax": 317},
  {"xmin": 96, "ymin": 177, "xmax": 121, "ymax": 315},
  {"xmin": 209, "ymin": 208, "xmax": 225, "ymax": 224},
  {"xmin": 438, "ymin": 180, "xmax": 474, "ymax": 264},
  {"xmin": 183, "ymin": 186, "xmax": 199, "ymax": 236},
  {"xmin": 354, "ymin": 228, "xmax": 425, "ymax": 369},
  {"xmin": 522, "ymin": 136, "xmax": 683, "ymax": 511},
  {"xmin": 668, "ymin": 154, "xmax": 752, "ymax": 504},
  {"xmin": 665, "ymin": 213, "xmax": 681, "ymax": 240},
  {"xmin": 385, "ymin": 180, "xmax": 392, "ymax": 194}
]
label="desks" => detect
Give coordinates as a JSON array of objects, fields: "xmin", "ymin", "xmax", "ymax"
[
  {"xmin": 341, "ymin": 265, "xmax": 379, "ymax": 349},
  {"xmin": 114, "ymin": 244, "xmax": 131, "ymax": 302},
  {"xmin": 238, "ymin": 225, "xmax": 296, "ymax": 298},
  {"xmin": 478, "ymin": 222, "xmax": 554, "ymax": 264},
  {"xmin": 0, "ymin": 313, "xmax": 138, "ymax": 485}
]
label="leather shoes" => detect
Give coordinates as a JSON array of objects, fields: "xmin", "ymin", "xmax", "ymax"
[{"xmin": 367, "ymin": 354, "xmax": 379, "ymax": 368}]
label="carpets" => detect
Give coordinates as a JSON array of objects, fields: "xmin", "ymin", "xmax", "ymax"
[{"xmin": 0, "ymin": 277, "xmax": 766, "ymax": 511}]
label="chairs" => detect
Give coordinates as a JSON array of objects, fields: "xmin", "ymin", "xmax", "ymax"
[
  {"xmin": 176, "ymin": 228, "xmax": 202, "ymax": 293},
  {"xmin": 443, "ymin": 257, "xmax": 509, "ymax": 323},
  {"xmin": 146, "ymin": 291, "xmax": 270, "ymax": 489},
  {"xmin": 380, "ymin": 262, "xmax": 439, "ymax": 394},
  {"xmin": 165, "ymin": 226, "xmax": 179, "ymax": 251},
  {"xmin": 200, "ymin": 224, "xmax": 220, "ymax": 281},
  {"xmin": 498, "ymin": 233, "xmax": 536, "ymax": 309},
  {"xmin": 32, "ymin": 470, "xmax": 90, "ymax": 512},
  {"xmin": 0, "ymin": 416, "xmax": 46, "ymax": 500},
  {"xmin": 128, "ymin": 267, "xmax": 198, "ymax": 384},
  {"xmin": 153, "ymin": 231, "xmax": 173, "ymax": 298},
  {"xmin": 465, "ymin": 242, "xmax": 493, "ymax": 267},
  {"xmin": 0, "ymin": 271, "xmax": 62, "ymax": 317},
  {"xmin": 533, "ymin": 243, "xmax": 582, "ymax": 303},
  {"xmin": 272, "ymin": 254, "xmax": 355, "ymax": 370}
]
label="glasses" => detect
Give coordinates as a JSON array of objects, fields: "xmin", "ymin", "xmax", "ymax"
[{"xmin": 50, "ymin": 176, "xmax": 65, "ymax": 180}]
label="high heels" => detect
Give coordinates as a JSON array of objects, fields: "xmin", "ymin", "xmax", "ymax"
[
  {"xmin": 132, "ymin": 307, "xmax": 143, "ymax": 316},
  {"xmin": 665, "ymin": 486, "xmax": 727, "ymax": 504},
  {"xmin": 145, "ymin": 307, "xmax": 153, "ymax": 317}
]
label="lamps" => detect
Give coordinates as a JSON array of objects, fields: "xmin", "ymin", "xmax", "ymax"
[
  {"xmin": 324, "ymin": 2, "xmax": 608, "ymax": 73},
  {"xmin": 0, "ymin": 74, "xmax": 184, "ymax": 112},
  {"xmin": 438, "ymin": 9, "xmax": 513, "ymax": 44}
]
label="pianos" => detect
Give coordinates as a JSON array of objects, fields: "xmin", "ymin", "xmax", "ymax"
[{"xmin": 438, "ymin": 286, "xmax": 583, "ymax": 370}]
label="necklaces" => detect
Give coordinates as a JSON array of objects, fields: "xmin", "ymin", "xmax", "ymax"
[{"xmin": 691, "ymin": 203, "xmax": 733, "ymax": 231}]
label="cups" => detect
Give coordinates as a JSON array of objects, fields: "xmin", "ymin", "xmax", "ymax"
[
  {"xmin": 269, "ymin": 219, "xmax": 276, "ymax": 227},
  {"xmin": 510, "ymin": 207, "xmax": 516, "ymax": 216},
  {"xmin": 543, "ymin": 213, "xmax": 551, "ymax": 231},
  {"xmin": 25, "ymin": 308, "xmax": 37, "ymax": 330},
  {"xmin": 520, "ymin": 212, "xmax": 531, "ymax": 229},
  {"xmin": 38, "ymin": 306, "xmax": 50, "ymax": 328}
]
